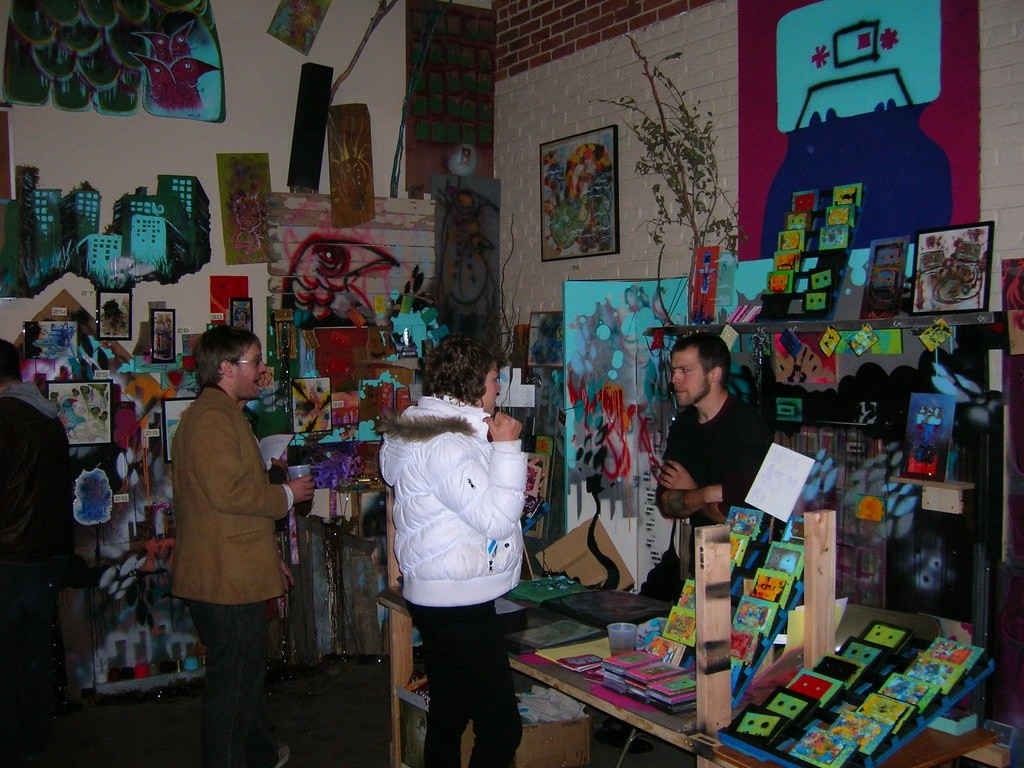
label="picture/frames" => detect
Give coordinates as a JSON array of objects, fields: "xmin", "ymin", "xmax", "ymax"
[
  {"xmin": 160, "ymin": 396, "xmax": 198, "ymax": 465},
  {"xmin": 45, "ymin": 379, "xmax": 115, "ymax": 447},
  {"xmin": 527, "ymin": 310, "xmax": 564, "ymax": 368},
  {"xmin": 95, "ymin": 288, "xmax": 134, "ymax": 342},
  {"xmin": 538, "ymin": 121, "xmax": 622, "ymax": 264},
  {"xmin": 148, "ymin": 308, "xmax": 177, "ymax": 366},
  {"xmin": 289, "ymin": 377, "xmax": 334, "ymax": 432},
  {"xmin": 907, "ymin": 218, "xmax": 995, "ymax": 315},
  {"xmin": 227, "ymin": 296, "xmax": 255, "ymax": 335}
]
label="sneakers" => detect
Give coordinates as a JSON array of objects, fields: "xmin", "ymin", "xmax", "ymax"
[{"xmin": 272, "ymin": 742, "xmax": 289, "ymax": 768}]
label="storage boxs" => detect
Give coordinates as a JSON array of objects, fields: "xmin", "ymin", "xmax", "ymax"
[
  {"xmin": 337, "ymin": 486, "xmax": 386, "ymax": 537},
  {"xmin": 461, "ymin": 708, "xmax": 592, "ymax": 768}
]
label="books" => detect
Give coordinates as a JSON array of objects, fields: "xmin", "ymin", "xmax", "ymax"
[
  {"xmin": 927, "ymin": 705, "xmax": 977, "ymax": 736},
  {"xmin": 556, "ymin": 654, "xmax": 604, "ymax": 682}
]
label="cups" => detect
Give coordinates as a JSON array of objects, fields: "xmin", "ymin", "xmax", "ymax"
[
  {"xmin": 287, "ymin": 464, "xmax": 310, "ymax": 481},
  {"xmin": 607, "ymin": 623, "xmax": 637, "ymax": 658}
]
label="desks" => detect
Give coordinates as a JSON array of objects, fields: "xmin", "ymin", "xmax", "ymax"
[{"xmin": 378, "ymin": 584, "xmax": 1012, "ymax": 768}]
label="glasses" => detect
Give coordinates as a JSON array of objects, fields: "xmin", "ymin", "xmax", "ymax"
[{"xmin": 236, "ymin": 356, "xmax": 262, "ymax": 367}]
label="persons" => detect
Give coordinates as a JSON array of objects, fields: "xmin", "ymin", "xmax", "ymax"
[
  {"xmin": 0, "ymin": 340, "xmax": 74, "ymax": 768},
  {"xmin": 627, "ymin": 332, "xmax": 776, "ymax": 626},
  {"xmin": 171, "ymin": 325, "xmax": 315, "ymax": 768},
  {"xmin": 379, "ymin": 332, "xmax": 528, "ymax": 768}
]
